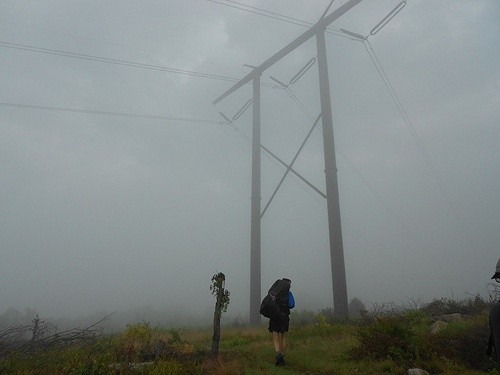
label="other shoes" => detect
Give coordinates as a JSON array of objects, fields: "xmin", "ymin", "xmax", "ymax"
[{"xmin": 275, "ymin": 352, "xmax": 285, "ymax": 366}]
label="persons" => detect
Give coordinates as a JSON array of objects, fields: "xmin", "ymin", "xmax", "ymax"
[
  {"xmin": 269, "ymin": 278, "xmax": 295, "ymax": 365},
  {"xmin": 488, "ymin": 258, "xmax": 500, "ymax": 375}
]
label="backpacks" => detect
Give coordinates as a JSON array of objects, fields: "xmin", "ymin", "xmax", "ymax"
[{"xmin": 260, "ymin": 279, "xmax": 291, "ymax": 320}]
